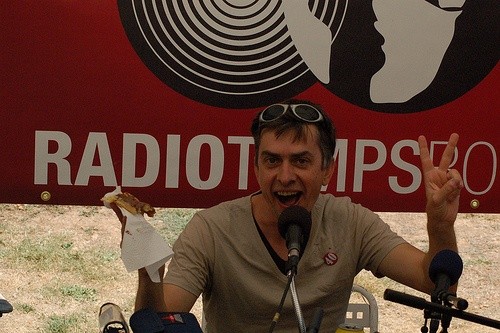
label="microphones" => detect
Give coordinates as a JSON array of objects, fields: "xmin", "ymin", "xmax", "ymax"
[
  {"xmin": 279, "ymin": 205, "xmax": 312, "ymax": 269},
  {"xmin": 428, "ymin": 249, "xmax": 463, "ymax": 296}
]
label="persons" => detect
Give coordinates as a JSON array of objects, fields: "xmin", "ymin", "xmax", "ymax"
[{"xmin": 110, "ymin": 100, "xmax": 463, "ymax": 333}]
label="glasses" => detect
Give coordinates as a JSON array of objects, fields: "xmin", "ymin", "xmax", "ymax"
[{"xmin": 258, "ymin": 103, "xmax": 329, "ymax": 131}]
